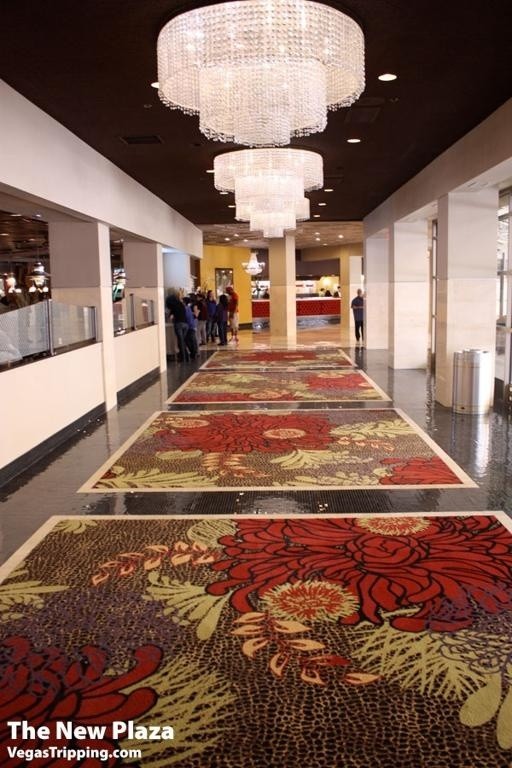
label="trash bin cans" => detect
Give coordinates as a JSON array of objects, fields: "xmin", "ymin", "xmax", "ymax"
[{"xmin": 453, "ymin": 349, "xmax": 494, "ymax": 415}]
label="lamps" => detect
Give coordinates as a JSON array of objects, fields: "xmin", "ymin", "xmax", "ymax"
[{"xmin": 241, "ymin": 248, "xmax": 266, "ymax": 274}]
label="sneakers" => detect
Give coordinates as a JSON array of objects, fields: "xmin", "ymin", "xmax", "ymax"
[
  {"xmin": 177, "ymin": 358, "xmax": 189, "ymax": 363},
  {"xmin": 190, "ymin": 353, "xmax": 200, "ymax": 358},
  {"xmin": 199, "ymin": 342, "xmax": 206, "ymax": 346},
  {"xmin": 229, "ymin": 337, "xmax": 239, "ymax": 341},
  {"xmin": 207, "ymin": 339, "xmax": 215, "ymax": 342},
  {"xmin": 218, "ymin": 343, "xmax": 227, "ymax": 346}
]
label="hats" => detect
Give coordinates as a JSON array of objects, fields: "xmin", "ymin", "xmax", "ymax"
[{"xmin": 226, "ymin": 287, "xmax": 232, "ymax": 292}]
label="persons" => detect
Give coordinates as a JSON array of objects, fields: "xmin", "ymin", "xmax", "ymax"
[
  {"xmin": 351, "ymin": 288, "xmax": 365, "ymax": 348},
  {"xmin": 263, "ymin": 289, "xmax": 270, "ymax": 299},
  {"xmin": 325, "ymin": 290, "xmax": 333, "ymax": 296},
  {"xmin": 164, "ymin": 284, "xmax": 240, "ymax": 363},
  {"xmin": 318, "ymin": 288, "xmax": 326, "ymax": 296},
  {"xmin": 334, "ymin": 285, "xmax": 342, "ymax": 297}
]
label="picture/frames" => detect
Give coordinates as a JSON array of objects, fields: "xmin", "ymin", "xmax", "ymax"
[{"xmin": 215, "ymin": 268, "xmax": 235, "ymax": 299}]
